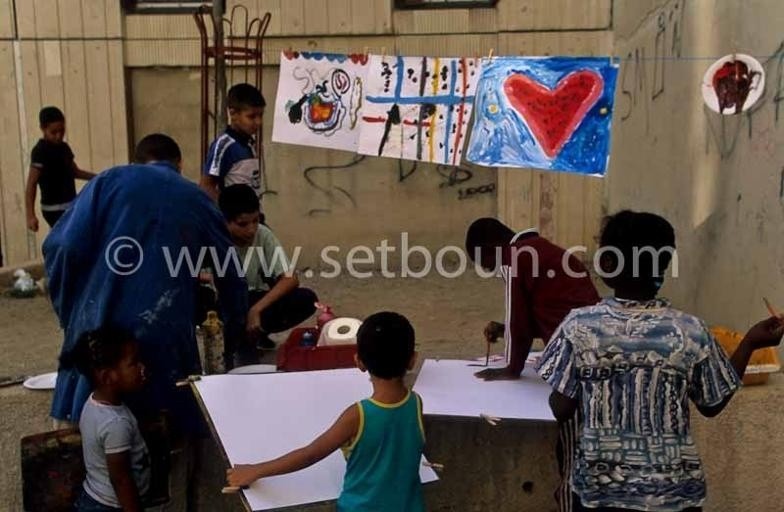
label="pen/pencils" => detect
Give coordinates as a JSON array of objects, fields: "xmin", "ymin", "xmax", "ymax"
[{"xmin": 763, "ymin": 296, "xmax": 781, "ymax": 320}]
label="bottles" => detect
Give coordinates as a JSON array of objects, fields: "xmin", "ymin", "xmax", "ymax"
[
  {"xmin": 317, "ymin": 305, "xmax": 336, "ymax": 337},
  {"xmin": 202, "ymin": 311, "xmax": 226, "ymax": 375},
  {"xmin": 194, "ymin": 324, "xmax": 206, "ymax": 376}
]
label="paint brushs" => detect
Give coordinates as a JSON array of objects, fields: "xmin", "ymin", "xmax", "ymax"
[{"xmin": 486, "ymin": 341, "xmax": 490, "ymax": 366}]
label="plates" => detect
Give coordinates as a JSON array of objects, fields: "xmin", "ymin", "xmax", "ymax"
[{"xmin": 22, "ymin": 370, "xmax": 59, "ymax": 390}]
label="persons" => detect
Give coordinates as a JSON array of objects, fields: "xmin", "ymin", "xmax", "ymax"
[
  {"xmin": 199, "ymin": 83, "xmax": 267, "ymax": 225},
  {"xmin": 466, "ymin": 218, "xmax": 603, "ymax": 487},
  {"xmin": 42, "ymin": 134, "xmax": 245, "ymax": 422},
  {"xmin": 24, "ymin": 106, "xmax": 98, "ymax": 231},
  {"xmin": 214, "ymin": 184, "xmax": 319, "ymax": 361},
  {"xmin": 226, "ymin": 311, "xmax": 426, "ymax": 511},
  {"xmin": 73, "ymin": 322, "xmax": 151, "ymax": 511},
  {"xmin": 534, "ymin": 209, "xmax": 783, "ymax": 511}
]
